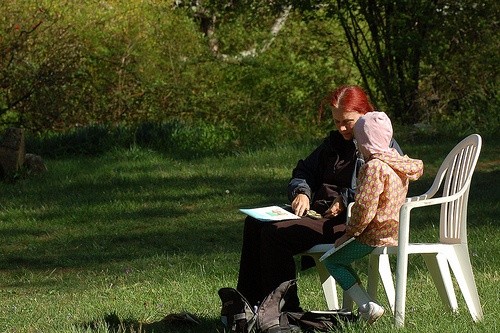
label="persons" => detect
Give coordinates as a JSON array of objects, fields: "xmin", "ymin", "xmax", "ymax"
[
  {"xmin": 326, "ymin": 111, "xmax": 409, "ymax": 326},
  {"xmin": 236, "ymin": 85, "xmax": 404, "ymax": 333}
]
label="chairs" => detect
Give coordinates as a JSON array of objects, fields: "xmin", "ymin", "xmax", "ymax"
[
  {"xmin": 347, "ymin": 134, "xmax": 484, "ymax": 328},
  {"xmin": 293, "ymin": 246, "xmax": 396, "ymax": 316}
]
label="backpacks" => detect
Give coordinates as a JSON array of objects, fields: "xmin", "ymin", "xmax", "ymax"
[{"xmin": 216, "ymin": 277, "xmax": 297, "ymax": 333}]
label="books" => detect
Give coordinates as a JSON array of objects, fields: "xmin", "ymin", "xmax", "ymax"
[{"xmin": 319, "ymin": 237, "xmax": 356, "ymax": 262}]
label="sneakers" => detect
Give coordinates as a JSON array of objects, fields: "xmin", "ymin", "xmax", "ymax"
[{"xmin": 355, "ymin": 300, "xmax": 384, "ymax": 327}]
